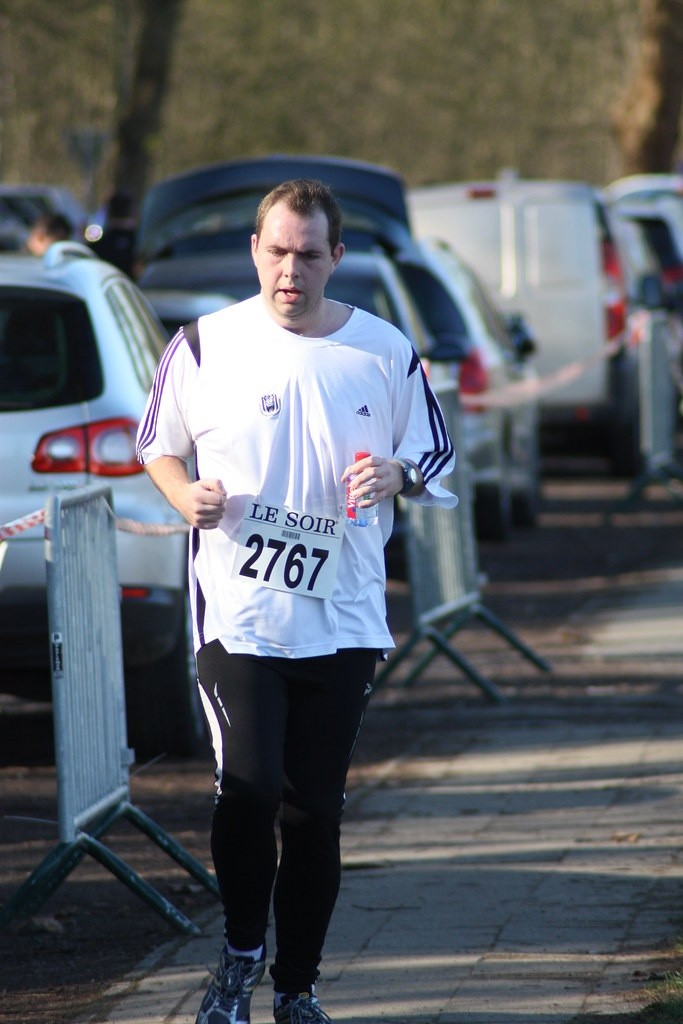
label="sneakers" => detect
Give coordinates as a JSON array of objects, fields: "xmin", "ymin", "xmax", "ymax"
[
  {"xmin": 273, "ymin": 984, "xmax": 332, "ymax": 1024},
  {"xmin": 195, "ymin": 940, "xmax": 266, "ymax": 1024}
]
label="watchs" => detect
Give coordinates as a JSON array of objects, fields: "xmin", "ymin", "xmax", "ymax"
[{"xmin": 393, "ymin": 459, "xmax": 417, "ymax": 494}]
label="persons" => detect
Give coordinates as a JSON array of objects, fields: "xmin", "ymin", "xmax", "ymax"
[
  {"xmin": 133, "ymin": 177, "xmax": 459, "ymax": 1024},
  {"xmin": 85, "ymin": 192, "xmax": 137, "ymax": 282},
  {"xmin": 17, "ymin": 210, "xmax": 74, "ymax": 257}
]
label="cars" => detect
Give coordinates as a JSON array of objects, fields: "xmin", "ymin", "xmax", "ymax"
[
  {"xmin": 124, "ymin": 246, "xmax": 454, "ymax": 588},
  {"xmin": 0, "ymin": 150, "xmax": 683, "ymax": 539}
]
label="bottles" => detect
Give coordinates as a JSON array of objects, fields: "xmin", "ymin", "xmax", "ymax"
[{"xmin": 346, "ymin": 452, "xmax": 378, "ymax": 527}]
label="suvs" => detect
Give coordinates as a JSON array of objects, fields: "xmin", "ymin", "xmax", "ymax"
[{"xmin": 0, "ymin": 242, "xmax": 197, "ymax": 719}]
